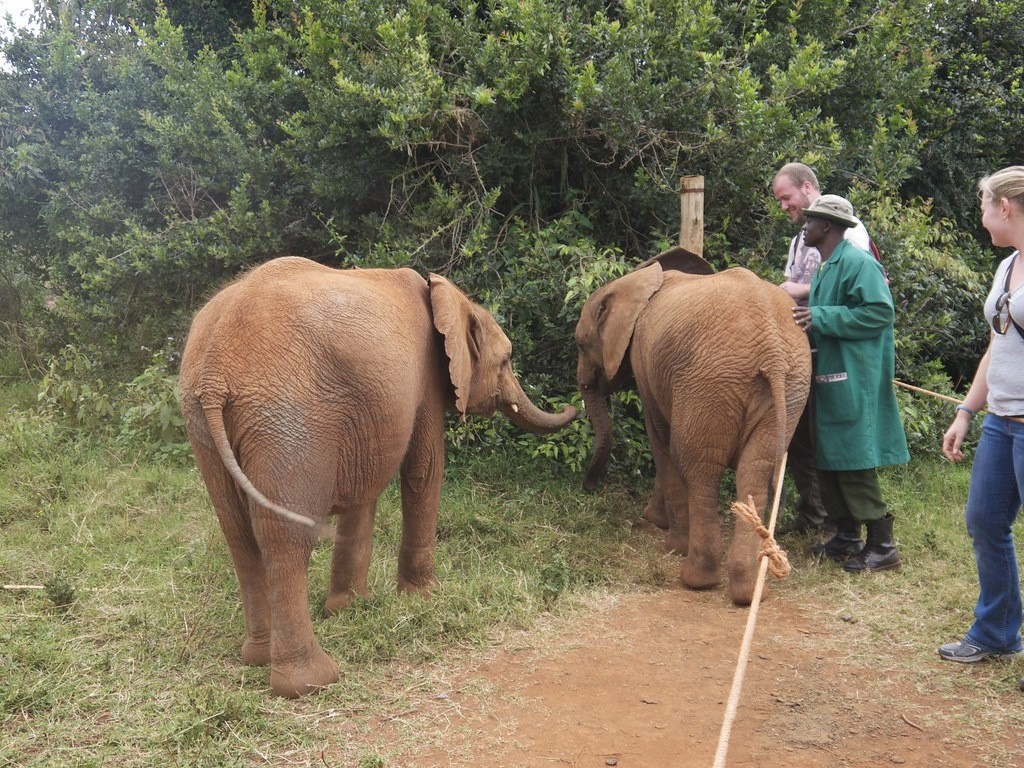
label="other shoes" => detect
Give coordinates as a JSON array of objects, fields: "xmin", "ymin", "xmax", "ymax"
[
  {"xmin": 806, "ymin": 523, "xmax": 837, "ymax": 548},
  {"xmin": 774, "ymin": 514, "xmax": 816, "ymax": 538}
]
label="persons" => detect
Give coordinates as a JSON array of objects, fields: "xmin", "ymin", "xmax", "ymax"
[
  {"xmin": 770, "ymin": 161, "xmax": 874, "ymax": 539},
  {"xmin": 791, "ymin": 194, "xmax": 911, "ymax": 572},
  {"xmin": 937, "ymin": 165, "xmax": 1024, "ymax": 664}
]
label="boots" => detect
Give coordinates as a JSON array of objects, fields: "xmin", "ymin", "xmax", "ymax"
[
  {"xmin": 844, "ymin": 514, "xmax": 904, "ymax": 572},
  {"xmin": 806, "ymin": 514, "xmax": 863, "ymax": 561}
]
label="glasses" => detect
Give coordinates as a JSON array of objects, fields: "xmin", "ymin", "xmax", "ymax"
[{"xmin": 993, "ymin": 292, "xmax": 1010, "ymax": 335}]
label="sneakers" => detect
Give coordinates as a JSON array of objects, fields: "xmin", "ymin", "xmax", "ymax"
[{"xmin": 939, "ymin": 637, "xmax": 1022, "ymax": 664}]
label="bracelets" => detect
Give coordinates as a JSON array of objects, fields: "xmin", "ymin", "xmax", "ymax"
[{"xmin": 956, "ymin": 405, "xmax": 974, "ymax": 417}]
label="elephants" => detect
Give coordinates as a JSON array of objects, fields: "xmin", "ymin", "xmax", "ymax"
[
  {"xmin": 575, "ymin": 245, "xmax": 814, "ymax": 606},
  {"xmin": 177, "ymin": 256, "xmax": 579, "ymax": 700}
]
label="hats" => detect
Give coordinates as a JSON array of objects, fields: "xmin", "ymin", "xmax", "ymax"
[{"xmin": 802, "ymin": 193, "xmax": 858, "ymax": 227}]
